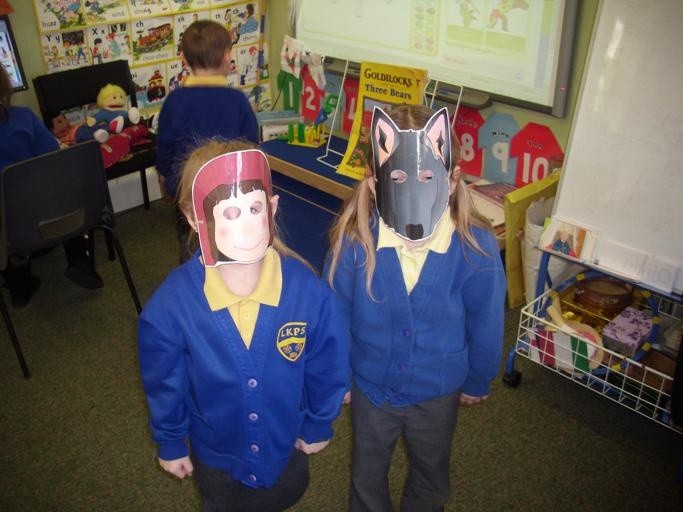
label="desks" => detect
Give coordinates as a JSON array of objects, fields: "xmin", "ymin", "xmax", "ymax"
[
  {"xmin": 103, "ymin": 129, "xmax": 162, "ymax": 209},
  {"xmin": 259, "ymin": 135, "xmax": 362, "ymax": 217}
]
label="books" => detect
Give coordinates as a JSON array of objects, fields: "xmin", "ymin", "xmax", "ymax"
[
  {"xmin": 538, "ymin": 218, "xmax": 591, "ymax": 262},
  {"xmin": 602, "ymin": 307, "xmax": 656, "ymax": 356},
  {"xmin": 594, "ymin": 240, "xmax": 677, "ymax": 295},
  {"xmin": 468, "ymin": 182, "xmax": 517, "ymax": 209}
]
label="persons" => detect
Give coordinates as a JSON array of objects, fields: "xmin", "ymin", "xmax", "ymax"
[
  {"xmin": 138, "ymin": 140, "xmax": 349, "ymax": 512},
  {"xmin": 552, "ymin": 231, "xmax": 571, "ymax": 253},
  {"xmin": 323, "ymin": 105, "xmax": 507, "ymax": 511},
  {"xmin": 0, "ymin": 66, "xmax": 103, "ymax": 308},
  {"xmin": 155, "ymin": 20, "xmax": 259, "ymax": 264}
]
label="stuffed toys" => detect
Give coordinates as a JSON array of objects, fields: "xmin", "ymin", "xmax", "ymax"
[
  {"xmin": 86, "ymin": 84, "xmax": 157, "ymax": 130},
  {"xmin": 47, "ymin": 112, "xmax": 124, "ymax": 149}
]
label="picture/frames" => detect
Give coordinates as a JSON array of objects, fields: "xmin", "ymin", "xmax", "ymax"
[{"xmin": 0, "ymin": 15, "xmax": 28, "ymax": 93}]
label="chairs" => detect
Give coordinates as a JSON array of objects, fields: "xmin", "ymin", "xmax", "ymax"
[{"xmin": 0, "ymin": 139, "xmax": 142, "ymax": 381}]
